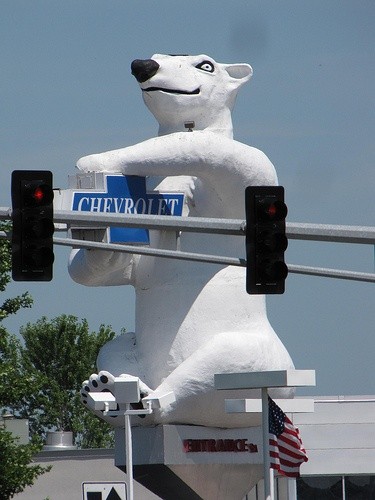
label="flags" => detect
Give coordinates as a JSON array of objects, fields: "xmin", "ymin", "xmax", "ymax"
[{"xmin": 265, "ymin": 395, "xmax": 309, "ymax": 483}]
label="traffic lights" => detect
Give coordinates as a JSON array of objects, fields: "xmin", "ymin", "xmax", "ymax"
[
  {"xmin": 244, "ymin": 185, "xmax": 288, "ymax": 295},
  {"xmin": 10, "ymin": 168, "xmax": 54, "ymax": 282}
]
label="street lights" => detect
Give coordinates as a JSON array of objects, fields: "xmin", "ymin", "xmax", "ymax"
[
  {"xmin": 86, "ymin": 375, "xmax": 177, "ymax": 500},
  {"xmin": 213, "ymin": 369, "xmax": 316, "ymax": 500}
]
